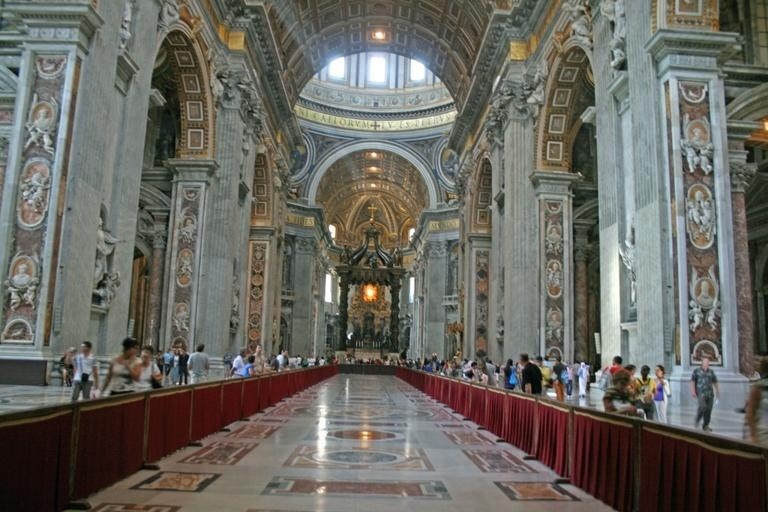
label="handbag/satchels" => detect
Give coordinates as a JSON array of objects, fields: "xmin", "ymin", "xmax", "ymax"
[
  {"xmin": 561, "ymin": 370, "xmax": 569, "ymax": 385},
  {"xmin": 82, "ymin": 373, "xmax": 89, "ymax": 382},
  {"xmin": 509, "ymin": 368, "xmax": 519, "ymax": 385}
]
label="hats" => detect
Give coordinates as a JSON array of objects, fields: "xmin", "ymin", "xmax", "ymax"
[{"xmin": 123, "ymin": 338, "xmax": 137, "ymax": 350}]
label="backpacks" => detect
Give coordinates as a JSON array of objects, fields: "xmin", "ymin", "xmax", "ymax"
[{"xmin": 637, "ymin": 378, "xmax": 654, "ymax": 404}]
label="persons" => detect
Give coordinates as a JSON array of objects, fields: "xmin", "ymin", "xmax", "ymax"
[
  {"xmin": 93, "ymin": 271, "xmax": 121, "ymax": 309},
  {"xmin": 561, "ymin": 2, "xmax": 595, "ymax": 39},
  {"xmin": 677, "ymin": 127, "xmax": 721, "ymax": 333},
  {"xmin": 741, "ymin": 356, "xmax": 767, "ymax": 449},
  {"xmin": 93, "ymin": 217, "xmax": 129, "ymax": 291},
  {"xmin": 621, "ymin": 238, "xmax": 637, "ymax": 305},
  {"xmin": 3, "ymin": 108, "xmax": 54, "ymax": 312},
  {"xmin": 689, "ymin": 355, "xmax": 721, "ymax": 430}
]
